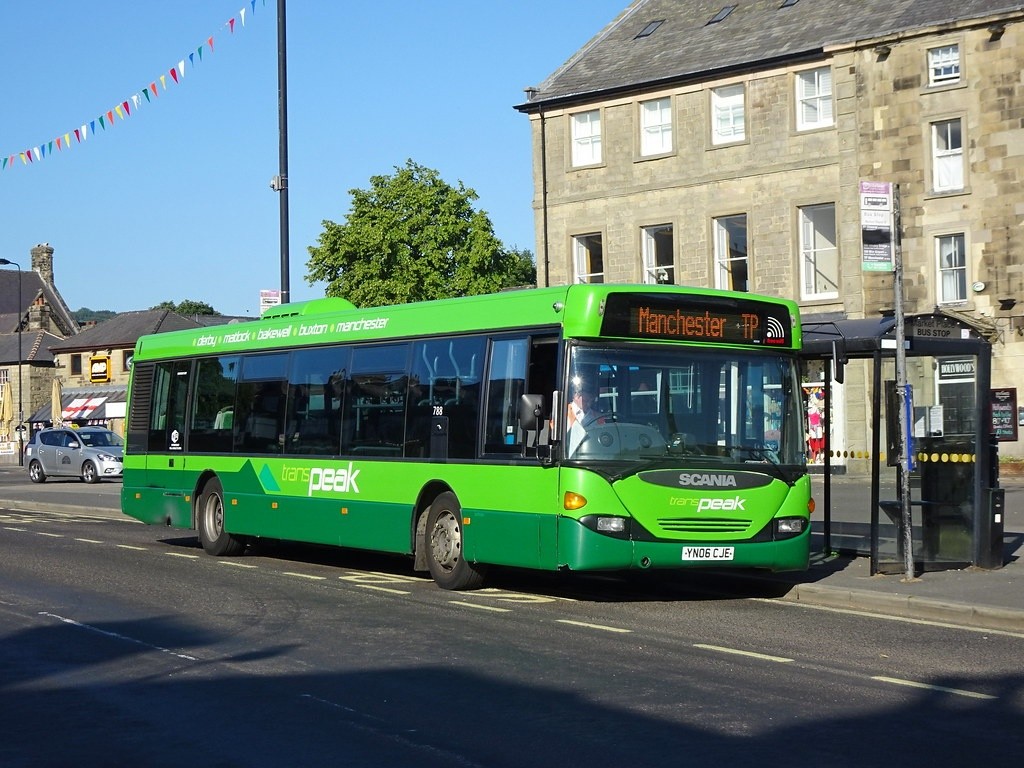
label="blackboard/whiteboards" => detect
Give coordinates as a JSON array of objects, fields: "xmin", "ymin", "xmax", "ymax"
[{"xmin": 991, "ymin": 387, "xmax": 1018, "ymax": 441}]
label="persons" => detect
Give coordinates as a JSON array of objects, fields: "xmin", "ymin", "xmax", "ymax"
[
  {"xmin": 549, "ymin": 386, "xmax": 604, "ymax": 432},
  {"xmin": 807, "ymin": 404, "xmax": 823, "ymax": 439}
]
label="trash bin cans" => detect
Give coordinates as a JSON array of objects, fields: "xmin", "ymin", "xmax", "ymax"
[{"xmin": 921, "ymin": 434, "xmax": 1006, "ymax": 570}]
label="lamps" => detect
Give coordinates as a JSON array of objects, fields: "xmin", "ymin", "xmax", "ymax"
[
  {"xmin": 999, "ymin": 298, "xmax": 1016, "ymax": 306},
  {"xmin": 105, "ymin": 348, "xmax": 111, "ymax": 352},
  {"xmin": 874, "ymin": 45, "xmax": 891, "ymax": 55},
  {"xmin": 89, "ymin": 350, "xmax": 97, "ymax": 353},
  {"xmin": 987, "ymin": 24, "xmax": 1006, "ymax": 33},
  {"xmin": 878, "ymin": 307, "xmax": 896, "ymax": 317}
]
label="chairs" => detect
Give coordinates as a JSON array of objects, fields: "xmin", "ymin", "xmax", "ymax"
[
  {"xmin": 213, "ymin": 406, "xmax": 233, "ymax": 430},
  {"xmin": 159, "ymin": 412, "xmax": 185, "ymax": 430},
  {"xmin": 92, "ymin": 433, "xmax": 110, "ymax": 446}
]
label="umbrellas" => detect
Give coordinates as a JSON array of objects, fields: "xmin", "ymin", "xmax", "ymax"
[
  {"xmin": 51, "ymin": 377, "xmax": 63, "ymax": 429},
  {"xmin": 0, "ymin": 382, "xmax": 14, "ymax": 435}
]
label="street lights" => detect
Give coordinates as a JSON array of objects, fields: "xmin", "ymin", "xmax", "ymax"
[{"xmin": 0, "ymin": 258, "xmax": 23, "ymax": 466}]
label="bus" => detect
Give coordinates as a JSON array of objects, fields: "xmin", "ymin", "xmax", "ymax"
[{"xmin": 116, "ymin": 292, "xmax": 849, "ymax": 592}]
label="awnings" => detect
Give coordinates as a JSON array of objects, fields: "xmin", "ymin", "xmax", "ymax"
[{"xmin": 24, "ymin": 390, "xmax": 127, "ymax": 422}]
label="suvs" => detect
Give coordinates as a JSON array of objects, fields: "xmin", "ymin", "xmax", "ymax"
[{"xmin": 23, "ymin": 426, "xmax": 126, "ymax": 484}]
